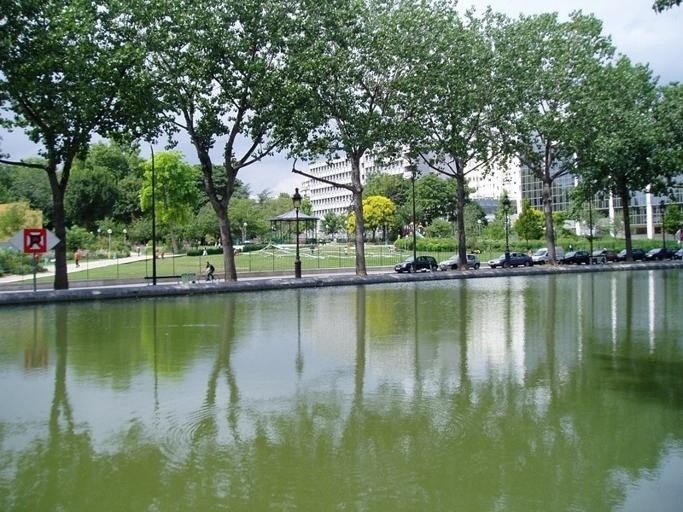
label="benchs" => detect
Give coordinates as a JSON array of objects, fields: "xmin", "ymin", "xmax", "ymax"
[{"xmin": 144, "ymin": 276, "xmax": 182, "ymax": 287}]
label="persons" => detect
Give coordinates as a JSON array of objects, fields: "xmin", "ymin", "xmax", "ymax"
[{"xmin": 205, "ymin": 261, "xmax": 214, "ymax": 282}]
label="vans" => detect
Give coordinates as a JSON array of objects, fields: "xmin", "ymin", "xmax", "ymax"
[{"xmin": 531, "ymin": 246, "xmax": 565, "ymax": 265}]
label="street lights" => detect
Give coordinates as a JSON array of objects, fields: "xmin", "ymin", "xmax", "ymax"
[
  {"xmin": 290, "ymin": 287, "xmax": 304, "ymax": 375},
  {"xmin": 291, "ymin": 186, "xmax": 305, "ymax": 280},
  {"xmin": 658, "ymin": 197, "xmax": 669, "ymax": 259},
  {"xmin": 243, "ymin": 223, "xmax": 247, "ymax": 246},
  {"xmin": 86, "ymin": 227, "xmax": 132, "ymax": 260},
  {"xmin": 502, "ymin": 193, "xmax": 512, "ymax": 269},
  {"xmin": 325, "ymin": 224, "xmax": 329, "ymax": 239}
]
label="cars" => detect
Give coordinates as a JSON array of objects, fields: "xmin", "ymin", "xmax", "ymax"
[
  {"xmin": 439, "ymin": 254, "xmax": 479, "ymax": 270},
  {"xmin": 487, "ymin": 250, "xmax": 534, "ymax": 268},
  {"xmin": 393, "ymin": 255, "xmax": 441, "ymax": 272},
  {"xmin": 563, "ymin": 247, "xmax": 682, "ymax": 265}
]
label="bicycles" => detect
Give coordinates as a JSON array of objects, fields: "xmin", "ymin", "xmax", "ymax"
[{"xmin": 203, "ymin": 272, "xmax": 222, "ymax": 284}]
